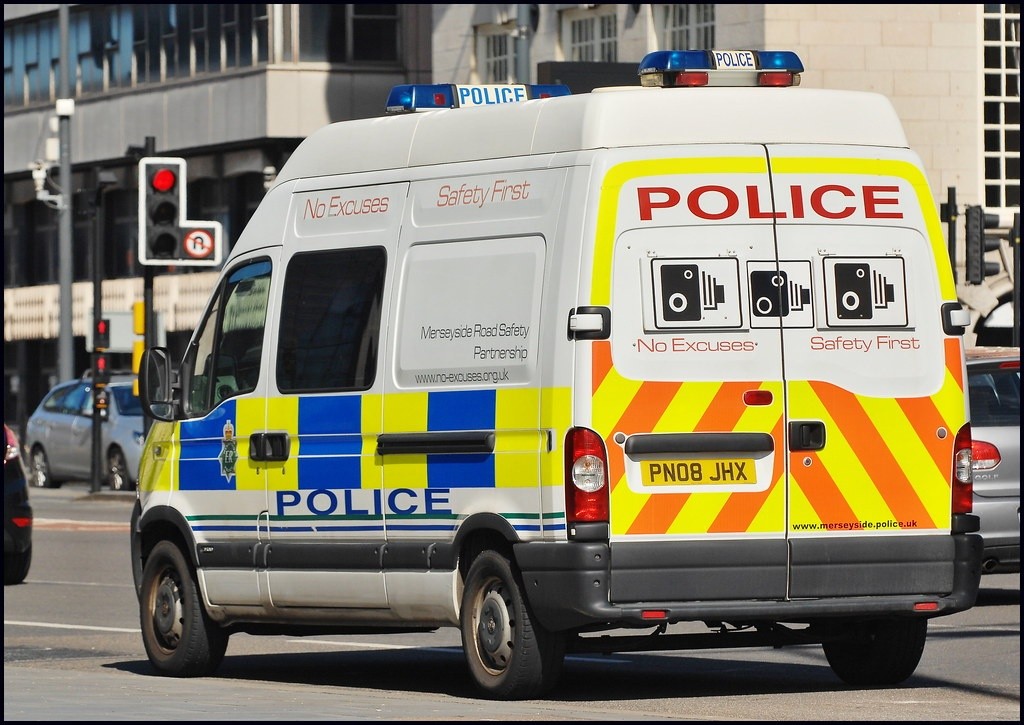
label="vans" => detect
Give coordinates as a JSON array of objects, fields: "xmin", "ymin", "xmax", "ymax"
[{"xmin": 131, "ymin": 50, "xmax": 986, "ymax": 700}]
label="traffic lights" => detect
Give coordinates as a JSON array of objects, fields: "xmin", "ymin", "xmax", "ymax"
[
  {"xmin": 91, "ymin": 352, "xmax": 110, "ymax": 385},
  {"xmin": 965, "ymin": 205, "xmax": 1003, "ymax": 288},
  {"xmin": 137, "ymin": 157, "xmax": 224, "ymax": 266},
  {"xmin": 95, "ymin": 318, "xmax": 111, "ymax": 350}
]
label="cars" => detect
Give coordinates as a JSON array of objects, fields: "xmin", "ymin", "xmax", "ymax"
[{"xmin": 4, "ymin": 422, "xmax": 34, "ymax": 585}]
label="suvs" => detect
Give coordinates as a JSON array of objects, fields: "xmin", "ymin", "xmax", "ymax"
[
  {"xmin": 962, "ymin": 345, "xmax": 1022, "ymax": 577},
  {"xmin": 23, "ymin": 365, "xmax": 150, "ymax": 492}
]
